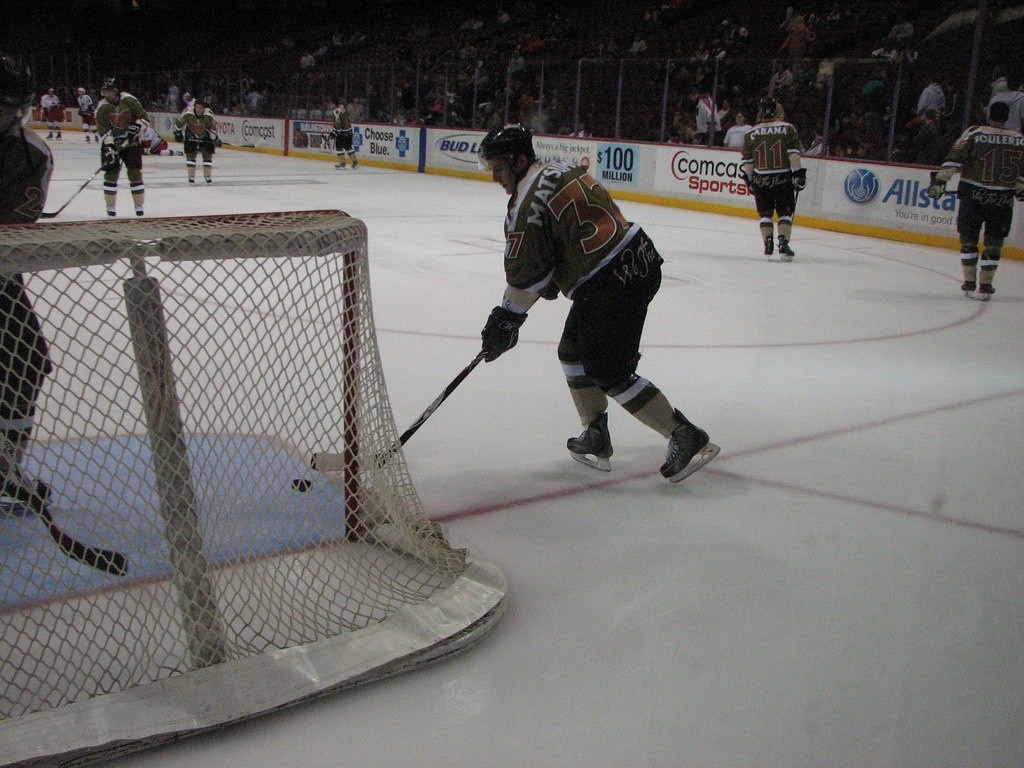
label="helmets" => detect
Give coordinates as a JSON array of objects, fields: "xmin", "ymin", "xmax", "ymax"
[
  {"xmin": 77, "ymin": 88, "xmax": 85, "ymax": 95},
  {"xmin": 757, "ymin": 98, "xmax": 779, "ymax": 119},
  {"xmin": 183, "ymin": 92, "xmax": 190, "ymax": 101},
  {"xmin": 0, "ymin": 54, "xmax": 37, "ymax": 110},
  {"xmin": 478, "ymin": 123, "xmax": 536, "ymax": 162},
  {"xmin": 100, "ymin": 77, "xmax": 119, "ymax": 96}
]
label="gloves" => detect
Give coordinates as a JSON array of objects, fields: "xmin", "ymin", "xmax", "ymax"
[
  {"xmin": 1014, "ymin": 179, "xmax": 1024, "ymax": 202},
  {"xmin": 126, "ymin": 123, "xmax": 142, "ymax": 145},
  {"xmin": 480, "ymin": 307, "xmax": 530, "ymax": 362},
  {"xmin": 328, "ymin": 130, "xmax": 338, "ymax": 139},
  {"xmin": 927, "ymin": 180, "xmax": 946, "ymax": 199},
  {"xmin": 103, "ymin": 143, "xmax": 118, "ymax": 167},
  {"xmin": 792, "ymin": 168, "xmax": 808, "ymax": 191},
  {"xmin": 540, "ymin": 281, "xmax": 559, "ymax": 301},
  {"xmin": 744, "ymin": 175, "xmax": 755, "ymax": 195}
]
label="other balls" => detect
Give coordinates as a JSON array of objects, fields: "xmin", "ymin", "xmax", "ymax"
[{"xmin": 290, "ymin": 478, "xmax": 313, "ymax": 493}]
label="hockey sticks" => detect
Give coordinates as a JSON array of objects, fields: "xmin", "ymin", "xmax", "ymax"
[
  {"xmin": 776, "ymin": 188, "xmax": 799, "ymax": 248},
  {"xmin": 39, "ymin": 137, "xmax": 134, "ymax": 218},
  {"xmin": 925, "ymin": 189, "xmax": 960, "ymax": 195},
  {"xmin": 183, "ymin": 139, "xmax": 256, "ymax": 149},
  {"xmin": 0, "ymin": 433, "xmax": 128, "ymax": 577},
  {"xmin": 307, "ymin": 292, "xmax": 552, "ymax": 472}
]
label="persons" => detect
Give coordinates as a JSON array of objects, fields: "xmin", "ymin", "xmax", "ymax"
[
  {"xmin": 0, "ymin": 51, "xmax": 55, "ymax": 509},
  {"xmin": 175, "ymin": 100, "xmax": 217, "ymax": 186},
  {"xmin": 142, "ymin": 127, "xmax": 184, "ymax": 156},
  {"xmin": 95, "ymin": 78, "xmax": 151, "ymax": 218},
  {"xmin": 927, "ymin": 102, "xmax": 1024, "ymax": 301},
  {"xmin": 41, "ymin": 88, "xmax": 62, "ymax": 139},
  {"xmin": 326, "ymin": 96, "xmax": 360, "ymax": 170},
  {"xmin": 181, "ymin": 92, "xmax": 222, "ymax": 152},
  {"xmin": 76, "ymin": 87, "xmax": 100, "ymax": 144},
  {"xmin": 0, "ymin": 0, "xmax": 1024, "ymax": 163},
  {"xmin": 479, "ymin": 121, "xmax": 720, "ymax": 484},
  {"xmin": 738, "ymin": 94, "xmax": 807, "ymax": 256}
]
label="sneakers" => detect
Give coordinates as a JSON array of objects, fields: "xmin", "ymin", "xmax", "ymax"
[
  {"xmin": 189, "ymin": 174, "xmax": 195, "ymax": 185},
  {"xmin": 567, "ymin": 412, "xmax": 613, "ymax": 470},
  {"xmin": 762, "ymin": 237, "xmax": 774, "ymax": 262},
  {"xmin": 205, "ymin": 176, "xmax": 212, "ymax": 186},
  {"xmin": 134, "ymin": 203, "xmax": 145, "ymax": 218},
  {"xmin": 0, "ymin": 467, "xmax": 54, "ymax": 511},
  {"xmin": 335, "ymin": 163, "xmax": 346, "ymax": 170},
  {"xmin": 106, "ymin": 205, "xmax": 116, "ymax": 219},
  {"xmin": 979, "ymin": 283, "xmax": 995, "ymax": 300},
  {"xmin": 777, "ymin": 235, "xmax": 794, "ymax": 262},
  {"xmin": 352, "ymin": 161, "xmax": 359, "ymax": 168},
  {"xmin": 961, "ymin": 281, "xmax": 977, "ymax": 298},
  {"xmin": 660, "ymin": 408, "xmax": 721, "ymax": 482}
]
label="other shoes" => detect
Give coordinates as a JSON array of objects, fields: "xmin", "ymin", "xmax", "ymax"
[
  {"xmin": 95, "ymin": 136, "xmax": 99, "ymax": 142},
  {"xmin": 177, "ymin": 150, "xmax": 184, "ymax": 156},
  {"xmin": 86, "ymin": 135, "xmax": 90, "ymax": 141},
  {"xmin": 56, "ymin": 132, "xmax": 61, "ymax": 138},
  {"xmin": 46, "ymin": 132, "xmax": 54, "ymax": 139}
]
